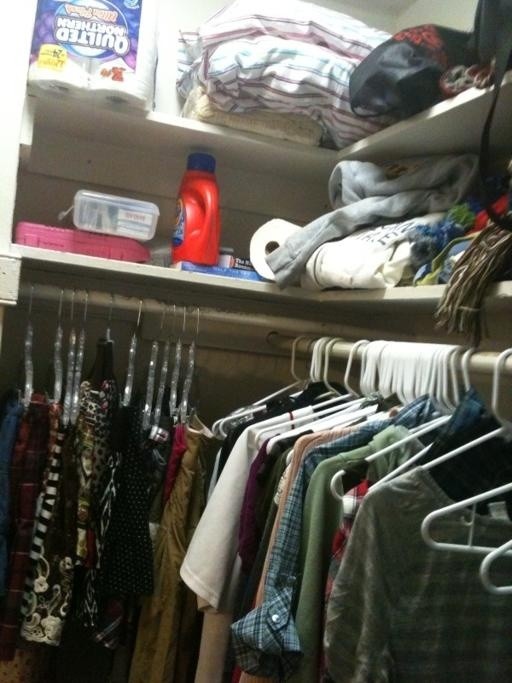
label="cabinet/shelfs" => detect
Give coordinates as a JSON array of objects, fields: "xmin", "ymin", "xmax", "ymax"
[{"xmin": 0, "ymin": 48, "xmax": 512, "ymax": 305}]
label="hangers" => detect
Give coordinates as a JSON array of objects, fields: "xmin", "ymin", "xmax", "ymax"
[
  {"xmin": 17, "ymin": 281, "xmax": 202, "ymax": 431},
  {"xmin": 211, "ymin": 334, "xmax": 511, "ymax": 596}
]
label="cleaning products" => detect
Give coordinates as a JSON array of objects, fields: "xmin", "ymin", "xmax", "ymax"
[{"xmin": 172, "ymin": 152, "xmax": 221, "ymax": 267}]
